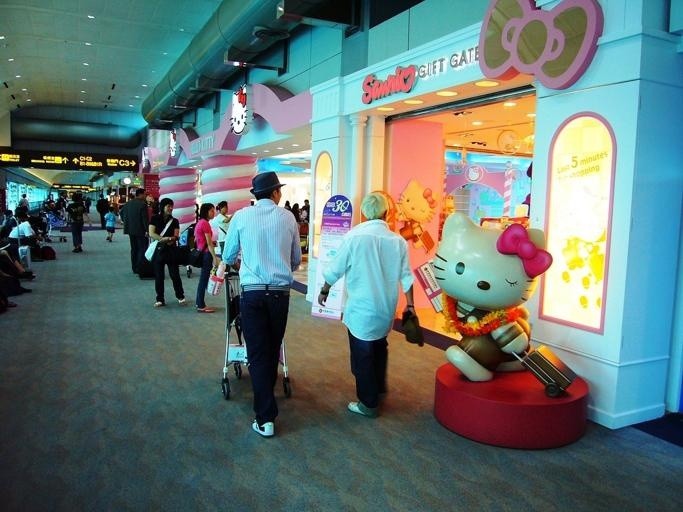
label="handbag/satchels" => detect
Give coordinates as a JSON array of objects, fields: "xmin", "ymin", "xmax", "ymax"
[
  {"xmin": 144, "ymin": 219, "xmax": 173, "ymax": 262},
  {"xmin": 189, "ymin": 241, "xmax": 208, "ymax": 268},
  {"xmin": 207, "ymin": 263, "xmax": 224, "ymax": 296}
]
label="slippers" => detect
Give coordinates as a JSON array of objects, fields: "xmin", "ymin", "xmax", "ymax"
[{"xmin": 197, "ymin": 307, "xmax": 214, "ymax": 312}]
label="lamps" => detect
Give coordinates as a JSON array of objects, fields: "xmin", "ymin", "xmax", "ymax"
[{"xmin": 121, "ymin": 178, "xmax": 132, "ymax": 186}]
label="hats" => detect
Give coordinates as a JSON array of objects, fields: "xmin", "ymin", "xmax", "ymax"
[{"xmin": 249, "ymin": 171, "xmax": 286, "ymax": 193}]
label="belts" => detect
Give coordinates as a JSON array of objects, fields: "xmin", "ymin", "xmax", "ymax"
[{"xmin": 242, "ymin": 285, "xmax": 290, "ymax": 292}]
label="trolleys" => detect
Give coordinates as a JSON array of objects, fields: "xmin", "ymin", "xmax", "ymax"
[
  {"xmin": 40, "ymin": 210, "xmax": 68, "ymax": 243},
  {"xmin": 222, "ymin": 273, "xmax": 292, "ymax": 400}
]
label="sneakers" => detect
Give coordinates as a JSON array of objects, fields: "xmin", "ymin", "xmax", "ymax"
[
  {"xmin": 154, "ymin": 302, "xmax": 162, "ymax": 307},
  {"xmin": 252, "ymin": 419, "xmax": 274, "ymax": 437},
  {"xmin": 347, "ymin": 401, "xmax": 367, "ymax": 415},
  {"xmin": 178, "ymin": 299, "xmax": 186, "ymax": 304}
]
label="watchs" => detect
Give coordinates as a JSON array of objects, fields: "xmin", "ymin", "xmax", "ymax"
[
  {"xmin": 169, "ymin": 237, "xmax": 171, "ymax": 241},
  {"xmin": 319, "ymin": 287, "xmax": 330, "ymax": 295}
]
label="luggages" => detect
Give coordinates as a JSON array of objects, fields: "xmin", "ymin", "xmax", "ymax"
[{"xmin": 513, "ymin": 344, "xmax": 577, "ymax": 398}]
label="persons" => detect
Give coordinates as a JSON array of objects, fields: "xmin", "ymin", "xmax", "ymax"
[
  {"xmin": 193, "ymin": 203, "xmax": 216, "ymax": 313},
  {"xmin": 104, "ymin": 207, "xmax": 116, "ymax": 242},
  {"xmin": 300, "ymin": 206, "xmax": 307, "ymax": 220},
  {"xmin": 317, "ymin": 194, "xmax": 416, "ymax": 417},
  {"xmin": 126, "ymin": 188, "xmax": 153, "ymax": 280},
  {"xmin": 222, "ymin": 171, "xmax": 301, "ymax": 438},
  {"xmin": 119, "ymin": 194, "xmax": 135, "ymax": 234},
  {"xmin": 148, "ymin": 198, "xmax": 186, "ymax": 307},
  {"xmin": 19, "ymin": 194, "xmax": 29, "ymax": 207},
  {"xmin": 216, "ymin": 201, "xmax": 233, "ymax": 260},
  {"xmin": 96, "ymin": 194, "xmax": 109, "ymax": 228},
  {"xmin": 8, "ymin": 218, "xmax": 43, "ymax": 247},
  {"xmin": 0, "ymin": 241, "xmax": 34, "ymax": 306},
  {"xmin": 304, "ymin": 199, "xmax": 310, "ymax": 223},
  {"xmin": 67, "ymin": 194, "xmax": 87, "ymax": 253},
  {"xmin": 291, "ymin": 203, "xmax": 301, "ymax": 221},
  {"xmin": 284, "ymin": 200, "xmax": 291, "ymax": 210}
]
